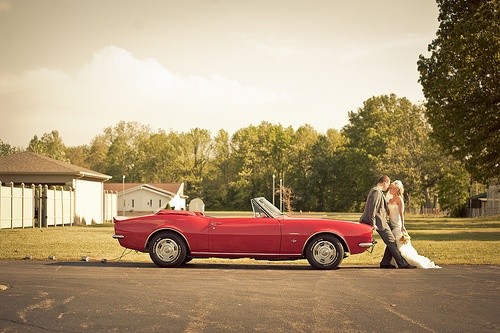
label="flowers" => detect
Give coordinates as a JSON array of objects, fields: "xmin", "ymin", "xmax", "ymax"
[{"xmin": 400, "ymin": 232, "xmax": 410, "ymax": 244}]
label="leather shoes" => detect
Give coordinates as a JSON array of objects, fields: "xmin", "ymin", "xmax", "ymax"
[
  {"xmin": 399, "ymin": 264, "xmax": 417, "ymax": 268},
  {"xmin": 380, "ymin": 262, "xmax": 396, "ymax": 268}
]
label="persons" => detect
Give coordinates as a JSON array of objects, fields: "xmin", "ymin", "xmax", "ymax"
[
  {"xmin": 360, "ymin": 175, "xmax": 417, "ymax": 269},
  {"xmin": 387, "ymin": 179, "xmax": 417, "ymax": 268}
]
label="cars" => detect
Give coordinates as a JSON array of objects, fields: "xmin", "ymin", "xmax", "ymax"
[{"xmin": 114, "ymin": 195, "xmax": 374, "ymax": 270}]
